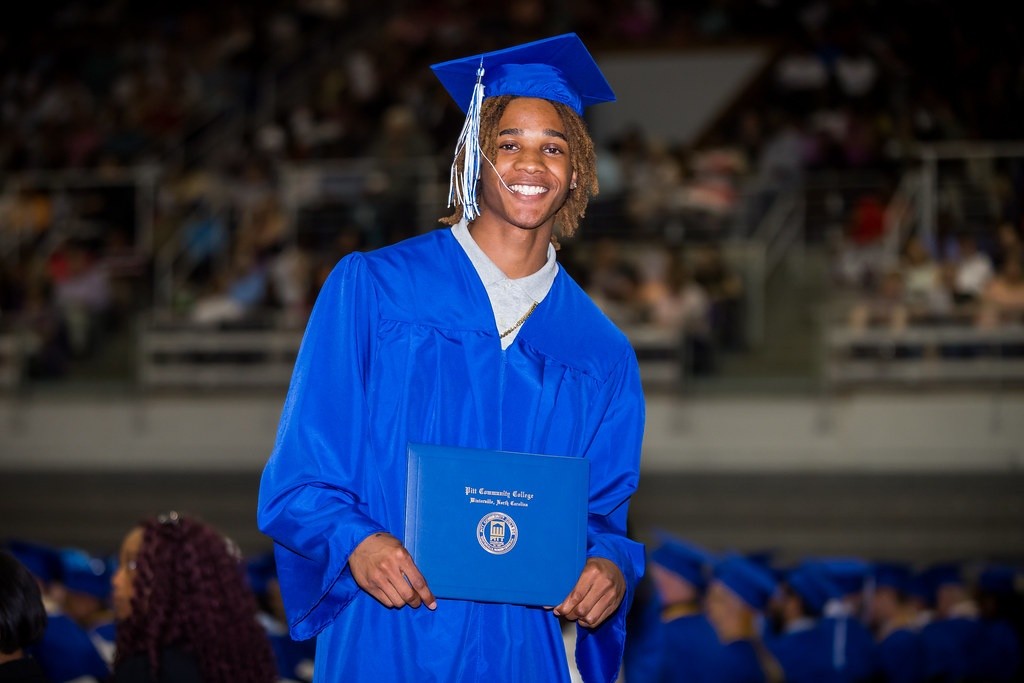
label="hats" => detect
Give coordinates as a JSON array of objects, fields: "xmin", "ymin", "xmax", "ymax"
[
  {"xmin": 433, "ymin": 33, "xmax": 615, "ymax": 225},
  {"xmin": 657, "ymin": 539, "xmax": 963, "ymax": 609},
  {"xmin": 18, "ymin": 534, "xmax": 111, "ymax": 609}
]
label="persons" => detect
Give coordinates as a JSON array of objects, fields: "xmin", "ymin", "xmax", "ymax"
[
  {"xmin": 1, "ymin": 121, "xmax": 1023, "ymax": 683},
  {"xmin": 258, "ymin": 33, "xmax": 647, "ymax": 683}
]
